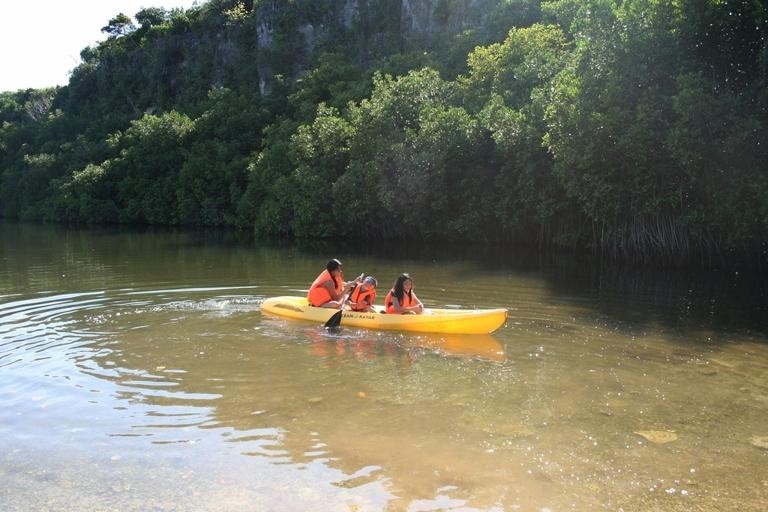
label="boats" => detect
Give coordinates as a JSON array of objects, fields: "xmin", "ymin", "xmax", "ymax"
[{"xmin": 258, "ymin": 294, "xmax": 509, "ymax": 336}]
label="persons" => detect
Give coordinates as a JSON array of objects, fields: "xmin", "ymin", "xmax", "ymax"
[
  {"xmin": 307, "ymin": 258, "xmax": 358, "ymax": 309},
  {"xmin": 344, "ymin": 276, "xmax": 377, "ymax": 312},
  {"xmin": 384, "ymin": 273, "xmax": 423, "ymax": 315}
]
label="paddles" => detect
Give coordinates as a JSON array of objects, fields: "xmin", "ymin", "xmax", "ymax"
[{"xmin": 325, "ymin": 274, "xmax": 364, "ymax": 332}]
